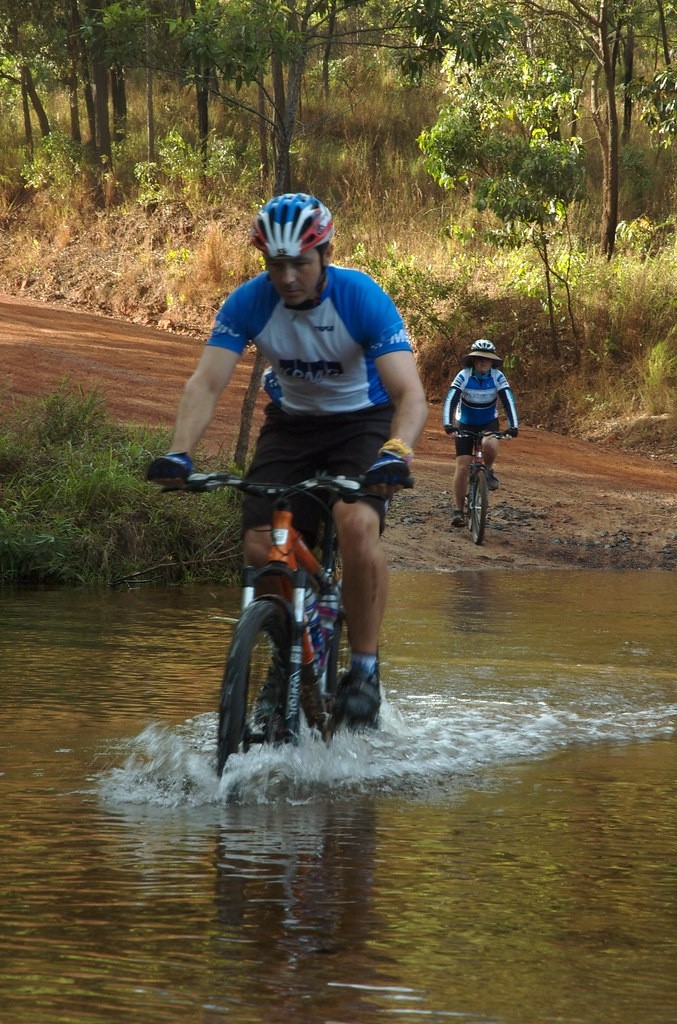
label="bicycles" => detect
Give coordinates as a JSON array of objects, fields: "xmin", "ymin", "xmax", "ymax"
[
  {"xmin": 451, "ymin": 429, "xmax": 512, "ymax": 545},
  {"xmin": 159, "ymin": 472, "xmax": 416, "ymax": 778}
]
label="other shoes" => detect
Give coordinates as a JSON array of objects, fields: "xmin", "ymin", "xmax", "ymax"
[
  {"xmin": 484, "ymin": 470, "xmax": 499, "ymax": 491},
  {"xmin": 452, "ymin": 510, "xmax": 464, "ymax": 527}
]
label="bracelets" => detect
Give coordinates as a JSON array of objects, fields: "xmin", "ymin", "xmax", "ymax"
[{"xmin": 378, "ymin": 439, "xmax": 413, "ymax": 466}]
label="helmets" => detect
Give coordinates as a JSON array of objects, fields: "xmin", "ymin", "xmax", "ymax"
[
  {"xmin": 250, "ymin": 193, "xmax": 334, "ymax": 259},
  {"xmin": 470, "ymin": 339, "xmax": 495, "ymax": 352}
]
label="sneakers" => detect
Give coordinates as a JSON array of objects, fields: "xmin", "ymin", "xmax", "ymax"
[{"xmin": 333, "ymin": 672, "xmax": 380, "ymax": 718}]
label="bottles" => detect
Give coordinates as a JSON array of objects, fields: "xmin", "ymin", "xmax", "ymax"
[
  {"xmin": 317, "ymin": 584, "xmax": 339, "ymax": 644},
  {"xmin": 305, "ymin": 580, "xmax": 326, "ymax": 654}
]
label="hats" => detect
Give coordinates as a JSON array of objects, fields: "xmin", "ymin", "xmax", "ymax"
[{"xmin": 463, "ymin": 352, "xmax": 503, "ymax": 369}]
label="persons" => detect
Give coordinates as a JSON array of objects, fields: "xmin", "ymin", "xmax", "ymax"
[
  {"xmin": 143, "ymin": 194, "xmax": 428, "ymax": 743},
  {"xmin": 443, "ymin": 339, "xmax": 518, "ymax": 528}
]
